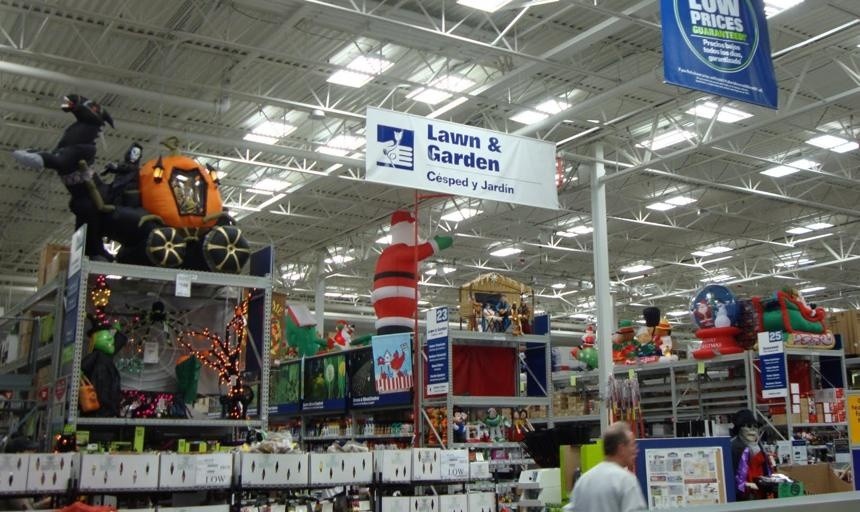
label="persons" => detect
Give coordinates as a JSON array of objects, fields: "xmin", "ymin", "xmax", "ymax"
[
  {"xmin": 557, "ymin": 419, "xmax": 648, "ymax": 511},
  {"xmin": 369, "ymin": 210, "xmax": 455, "ymax": 334},
  {"xmin": 729, "ymin": 408, "xmax": 784, "ymax": 500}
]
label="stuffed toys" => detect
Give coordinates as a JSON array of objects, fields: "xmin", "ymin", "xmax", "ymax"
[
  {"xmin": 472, "ymin": 294, "xmax": 532, "ymax": 335},
  {"xmin": 319, "ymin": 320, "xmax": 356, "ymax": 353},
  {"xmin": 423, "ymin": 404, "xmax": 534, "ymax": 443},
  {"xmin": 570, "ymin": 307, "xmax": 679, "ymax": 369},
  {"xmin": 684, "ymin": 280, "xmax": 745, "ymax": 357}
]
